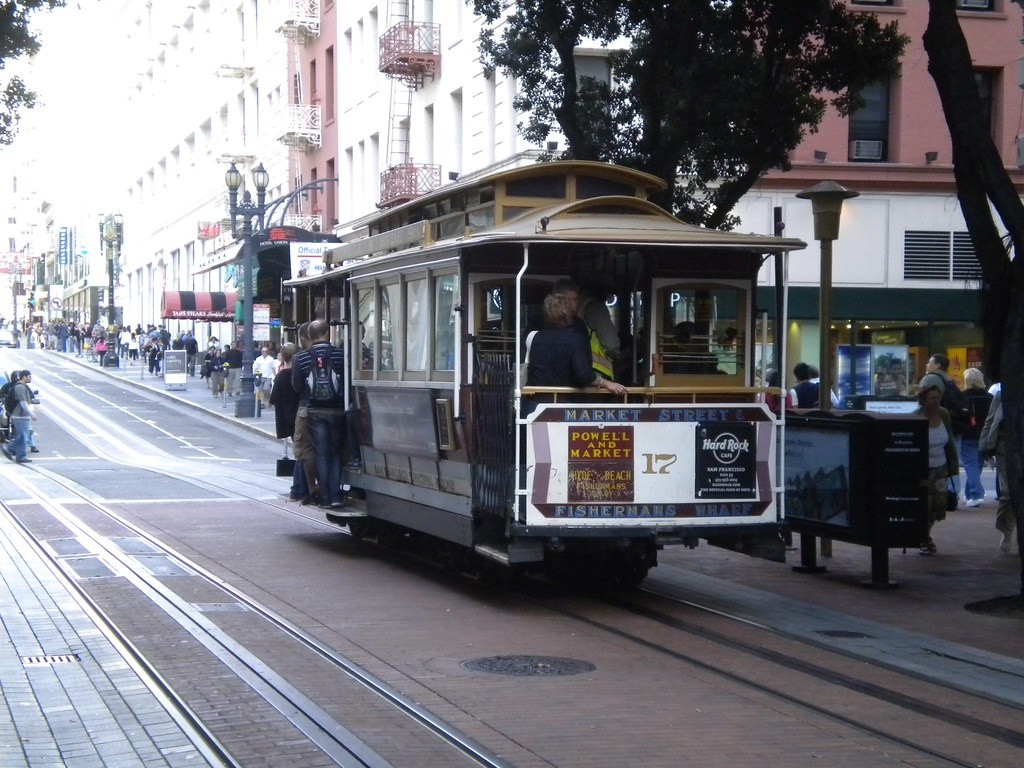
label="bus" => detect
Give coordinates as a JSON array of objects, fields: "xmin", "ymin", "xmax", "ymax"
[{"xmin": 276, "ymin": 159, "xmax": 802, "ymax": 601}]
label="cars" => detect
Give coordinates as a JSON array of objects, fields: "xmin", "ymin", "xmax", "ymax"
[
  {"xmin": 0, "ymin": 369, "xmax": 13, "ymax": 440},
  {"xmin": 0, "ymin": 323, "xmax": 22, "ymax": 347}
]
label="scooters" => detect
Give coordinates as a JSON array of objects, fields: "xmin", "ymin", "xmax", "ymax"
[{"xmin": 0, "ymin": 389, "xmax": 39, "ymax": 456}]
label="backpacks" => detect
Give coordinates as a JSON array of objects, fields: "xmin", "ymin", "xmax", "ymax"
[
  {"xmin": 927, "ymin": 371, "xmax": 975, "ymax": 436},
  {"xmin": 4, "ymin": 383, "xmax": 24, "ymax": 413},
  {"xmin": 305, "ymin": 345, "xmax": 344, "ymax": 407}
]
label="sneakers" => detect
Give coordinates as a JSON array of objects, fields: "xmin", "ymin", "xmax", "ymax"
[
  {"xmin": 1000, "ymin": 532, "xmax": 1012, "ymax": 551},
  {"xmin": 964, "ymin": 495, "xmax": 984, "ymax": 507}
]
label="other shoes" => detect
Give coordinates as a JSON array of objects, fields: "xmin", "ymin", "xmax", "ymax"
[
  {"xmin": 16, "ymin": 457, "xmax": 33, "ymax": 462},
  {"xmin": 2, "ymin": 446, "xmax": 13, "ymax": 460},
  {"xmin": 31, "ymin": 446, "xmax": 39, "ymax": 452},
  {"xmin": 319, "ymin": 494, "xmax": 344, "ymax": 509}
]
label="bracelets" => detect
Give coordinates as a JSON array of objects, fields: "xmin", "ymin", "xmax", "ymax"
[{"xmin": 597, "ymin": 375, "xmax": 603, "ymax": 388}]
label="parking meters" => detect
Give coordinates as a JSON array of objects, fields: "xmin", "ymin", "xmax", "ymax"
[
  {"xmin": 123, "ymin": 341, "xmax": 129, "ymax": 373},
  {"xmin": 254, "ymin": 370, "xmax": 262, "ymax": 417},
  {"xmin": 139, "ymin": 347, "xmax": 146, "ymax": 379},
  {"xmin": 222, "ymin": 361, "xmax": 230, "ymax": 408}
]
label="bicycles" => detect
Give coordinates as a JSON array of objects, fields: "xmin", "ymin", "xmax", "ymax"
[{"xmin": 85, "ymin": 340, "xmax": 96, "ymax": 362}]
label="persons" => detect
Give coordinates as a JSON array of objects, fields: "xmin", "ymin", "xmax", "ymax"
[
  {"xmin": 753, "ymin": 362, "xmax": 840, "ymax": 413},
  {"xmin": 268, "ymin": 319, "xmax": 369, "ymax": 509},
  {"xmin": 874, "ymin": 357, "xmax": 906, "ymax": 398},
  {"xmin": 201, "ymin": 333, "xmax": 287, "ymax": 409},
  {"xmin": 511, "ymin": 270, "xmax": 630, "ymax": 464},
  {"xmin": 664, "ymin": 320, "xmax": 740, "ymax": 375},
  {"xmin": 0, "ymin": 370, "xmax": 39, "ymax": 463},
  {"xmin": 0, "ymin": 312, "xmax": 199, "ymax": 378},
  {"xmin": 979, "ymin": 389, "xmax": 1016, "ymax": 552},
  {"xmin": 910, "ymin": 353, "xmax": 1001, "ymax": 507},
  {"xmin": 910, "ymin": 386, "xmax": 960, "ymax": 556}
]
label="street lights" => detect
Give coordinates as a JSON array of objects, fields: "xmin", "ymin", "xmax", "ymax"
[
  {"xmin": 98, "ymin": 213, "xmax": 123, "ymax": 368},
  {"xmin": 793, "ymin": 181, "xmax": 861, "ymax": 557},
  {"xmin": 224, "ymin": 160, "xmax": 269, "ymax": 417}
]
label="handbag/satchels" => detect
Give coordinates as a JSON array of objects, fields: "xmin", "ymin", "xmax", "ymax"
[
  {"xmin": 510, "ymin": 363, "xmax": 528, "ymax": 388},
  {"xmin": 945, "ymin": 474, "xmax": 959, "ymax": 512}
]
